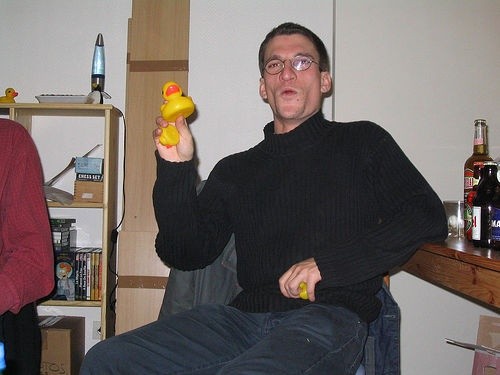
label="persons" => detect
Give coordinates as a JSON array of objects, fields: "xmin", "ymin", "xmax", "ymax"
[
  {"xmin": 0, "ymin": 118, "xmax": 54, "ymax": 375},
  {"xmin": 79, "ymin": 22, "xmax": 448, "ymax": 374}
]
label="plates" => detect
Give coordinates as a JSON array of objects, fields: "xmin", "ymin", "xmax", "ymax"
[{"xmin": 36, "ymin": 95, "xmax": 91, "ymax": 103}]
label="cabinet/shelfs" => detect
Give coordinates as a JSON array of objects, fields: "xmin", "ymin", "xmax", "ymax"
[{"xmin": 0, "ymin": 104, "xmax": 122, "ymax": 341}]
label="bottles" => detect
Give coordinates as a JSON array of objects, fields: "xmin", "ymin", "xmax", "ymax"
[
  {"xmin": 464, "ymin": 119, "xmax": 494, "ymax": 237},
  {"xmin": 466, "ymin": 161, "xmax": 485, "ymax": 241},
  {"xmin": 471, "ymin": 161, "xmax": 498, "ymax": 247},
  {"xmin": 491, "ymin": 182, "xmax": 500, "ymax": 251}
]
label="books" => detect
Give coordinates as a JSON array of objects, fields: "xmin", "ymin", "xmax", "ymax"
[{"xmin": 50, "ymin": 219, "xmax": 102, "ymax": 300}]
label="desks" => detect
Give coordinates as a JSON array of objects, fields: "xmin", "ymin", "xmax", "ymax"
[{"xmin": 397, "ymin": 231, "xmax": 500, "ymax": 315}]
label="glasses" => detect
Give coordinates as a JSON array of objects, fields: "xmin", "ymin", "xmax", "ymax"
[{"xmin": 261, "ymin": 55, "xmax": 322, "ymax": 78}]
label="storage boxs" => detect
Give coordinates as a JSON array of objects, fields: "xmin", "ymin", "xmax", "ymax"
[
  {"xmin": 73, "ymin": 180, "xmax": 103, "ymax": 203},
  {"xmin": 36, "ymin": 314, "xmax": 86, "ymax": 375},
  {"xmin": 444, "ymin": 316, "xmax": 500, "ymax": 375}
]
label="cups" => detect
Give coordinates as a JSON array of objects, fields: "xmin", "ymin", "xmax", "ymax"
[{"xmin": 444, "ymin": 201, "xmax": 466, "ymax": 238}]
